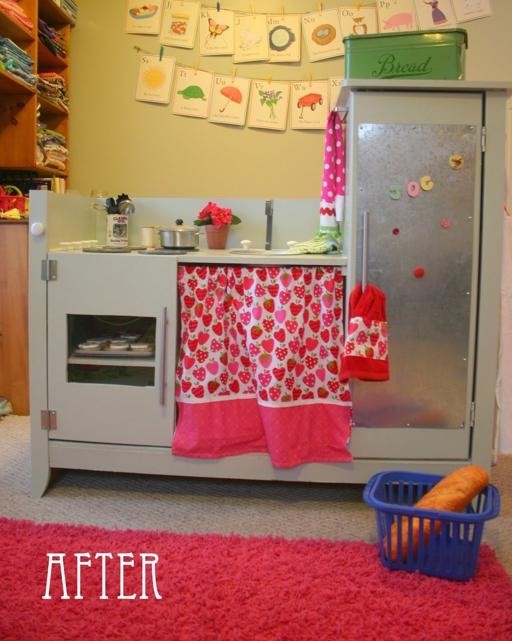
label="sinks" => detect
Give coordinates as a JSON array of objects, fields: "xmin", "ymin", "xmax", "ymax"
[{"xmin": 231, "ymin": 248, "xmax": 305, "ymax": 256}]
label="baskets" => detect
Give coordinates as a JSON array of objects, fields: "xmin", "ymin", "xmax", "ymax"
[{"xmin": 364, "ymin": 471, "xmax": 499, "ymax": 580}]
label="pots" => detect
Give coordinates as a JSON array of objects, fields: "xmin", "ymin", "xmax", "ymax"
[{"xmin": 157, "ymin": 219, "xmax": 205, "ymax": 248}]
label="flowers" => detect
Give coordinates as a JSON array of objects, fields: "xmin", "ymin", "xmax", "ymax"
[{"xmin": 193, "ymin": 202, "xmax": 241, "ymax": 230}]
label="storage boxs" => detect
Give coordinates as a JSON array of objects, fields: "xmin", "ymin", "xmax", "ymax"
[{"xmin": 342, "ymin": 28, "xmax": 468, "ymax": 80}]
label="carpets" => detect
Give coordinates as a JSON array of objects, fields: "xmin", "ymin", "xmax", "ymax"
[{"xmin": 0, "ymin": 518, "xmax": 511, "ymax": 641}]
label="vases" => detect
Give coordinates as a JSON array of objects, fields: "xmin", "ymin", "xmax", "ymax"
[{"xmin": 206, "ymin": 225, "xmax": 229, "ymax": 249}]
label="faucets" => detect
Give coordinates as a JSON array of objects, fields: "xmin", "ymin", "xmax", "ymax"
[{"xmin": 264, "ymin": 200, "xmax": 273, "ymax": 250}]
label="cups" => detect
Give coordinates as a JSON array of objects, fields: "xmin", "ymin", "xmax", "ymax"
[
  {"xmin": 105, "ymin": 214, "xmax": 129, "ymax": 247},
  {"xmin": 142, "ymin": 225, "xmax": 158, "ymax": 248}
]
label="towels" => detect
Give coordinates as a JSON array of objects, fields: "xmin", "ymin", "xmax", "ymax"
[
  {"xmin": 319, "ymin": 112, "xmax": 345, "ymax": 226},
  {"xmin": 339, "ymin": 282, "xmax": 389, "ymax": 385}
]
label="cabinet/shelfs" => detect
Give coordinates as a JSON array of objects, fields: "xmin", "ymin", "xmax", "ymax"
[{"xmin": 0, "ymin": 0, "xmax": 75, "ymax": 415}]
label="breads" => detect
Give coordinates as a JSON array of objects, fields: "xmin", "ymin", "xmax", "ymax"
[{"xmin": 382, "ymin": 466, "xmax": 490, "ymax": 562}]
